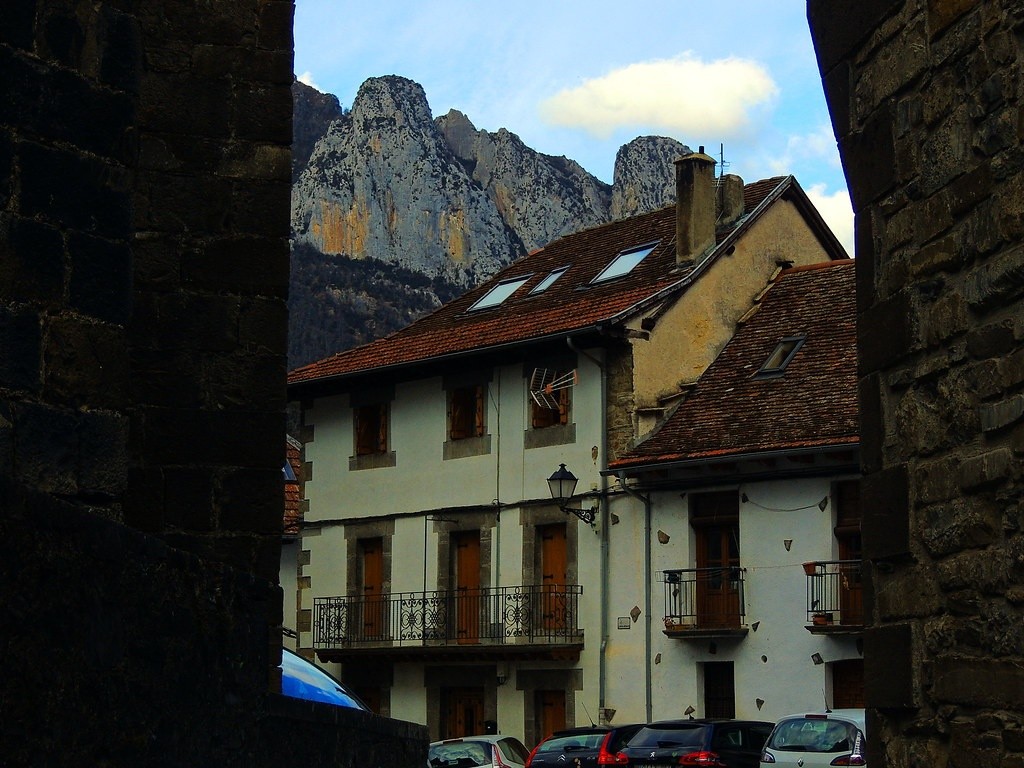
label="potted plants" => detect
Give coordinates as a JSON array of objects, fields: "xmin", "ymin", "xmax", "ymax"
[{"xmin": 812, "ymin": 612, "xmax": 834, "ymax": 625}]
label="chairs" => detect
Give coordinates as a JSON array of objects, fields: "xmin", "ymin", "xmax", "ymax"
[{"xmin": 564, "ymin": 740, "xmax": 580, "ymax": 746}]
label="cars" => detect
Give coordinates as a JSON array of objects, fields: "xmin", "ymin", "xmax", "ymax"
[
  {"xmin": 426, "ymin": 735, "xmax": 530, "ymax": 768},
  {"xmin": 614, "ymin": 717, "xmax": 778, "ymax": 768},
  {"xmin": 525, "ymin": 723, "xmax": 650, "ymax": 768},
  {"xmin": 759, "ymin": 708, "xmax": 868, "ymax": 768}
]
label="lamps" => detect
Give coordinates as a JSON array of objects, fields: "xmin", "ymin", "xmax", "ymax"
[{"xmin": 547, "ymin": 464, "xmax": 598, "ymax": 535}]
